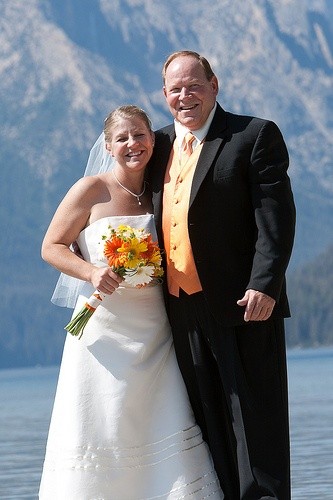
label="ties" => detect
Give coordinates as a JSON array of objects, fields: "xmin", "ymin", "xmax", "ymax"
[{"xmin": 181, "ymin": 132, "xmax": 196, "ymax": 171}]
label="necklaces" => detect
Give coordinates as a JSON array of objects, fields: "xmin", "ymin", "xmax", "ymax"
[{"xmin": 112, "ymin": 167, "xmax": 146, "ymax": 206}]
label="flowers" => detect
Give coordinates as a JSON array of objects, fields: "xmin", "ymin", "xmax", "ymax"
[{"xmin": 63, "ymin": 223, "xmax": 164, "ymax": 341}]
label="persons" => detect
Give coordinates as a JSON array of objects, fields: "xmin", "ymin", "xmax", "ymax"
[
  {"xmin": 39, "ymin": 104, "xmax": 225, "ymax": 500},
  {"xmin": 144, "ymin": 50, "xmax": 296, "ymax": 500}
]
